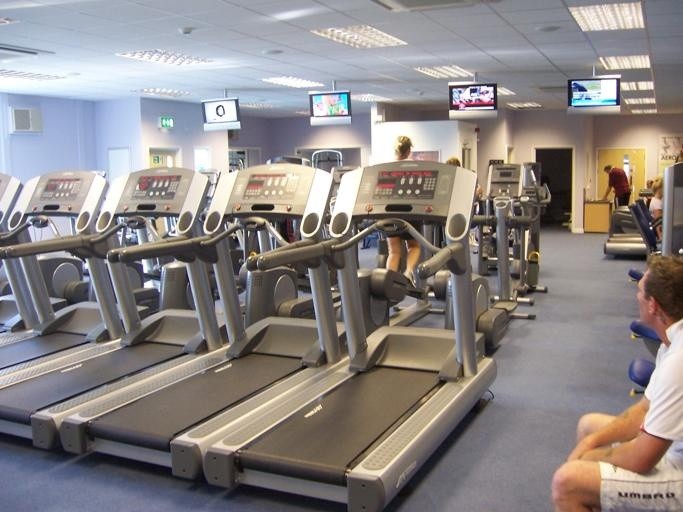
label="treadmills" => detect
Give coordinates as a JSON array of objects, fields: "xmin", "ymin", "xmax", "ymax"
[
  {"xmin": 0, "ymin": 170, "xmax": 125, "ymax": 388},
  {"xmin": 204, "ymin": 160, "xmax": 496, "ymax": 512},
  {"xmin": 58, "ymin": 162, "xmax": 346, "ymax": 480},
  {"xmin": 1, "ymin": 166, "xmax": 229, "ymax": 455}
]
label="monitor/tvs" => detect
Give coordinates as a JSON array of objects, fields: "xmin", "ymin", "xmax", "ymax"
[
  {"xmin": 308, "ymin": 88, "xmax": 352, "ymax": 127},
  {"xmin": 567, "ymin": 78, "xmax": 622, "ymax": 114},
  {"xmin": 201, "ymin": 97, "xmax": 242, "ymax": 131},
  {"xmin": 448, "ymin": 83, "xmax": 498, "ymax": 121}
]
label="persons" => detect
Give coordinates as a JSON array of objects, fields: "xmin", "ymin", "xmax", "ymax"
[
  {"xmin": 602, "ymin": 165, "xmax": 633, "ymax": 204},
  {"xmin": 216, "ymin": 105, "xmax": 225, "ymax": 122},
  {"xmin": 648, "ymin": 177, "xmax": 668, "ymax": 240},
  {"xmin": 551, "ymin": 254, "xmax": 683, "ymax": 511},
  {"xmin": 446, "ymin": 158, "xmax": 482, "ymax": 246},
  {"xmin": 380, "ymin": 137, "xmax": 422, "ymax": 288}
]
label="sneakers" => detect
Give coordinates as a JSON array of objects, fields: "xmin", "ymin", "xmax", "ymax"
[{"xmin": 403, "ymin": 271, "xmax": 417, "ymax": 290}]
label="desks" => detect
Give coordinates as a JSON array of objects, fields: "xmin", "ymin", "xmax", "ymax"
[{"xmin": 583, "ymin": 201, "xmax": 613, "ymax": 232}]
